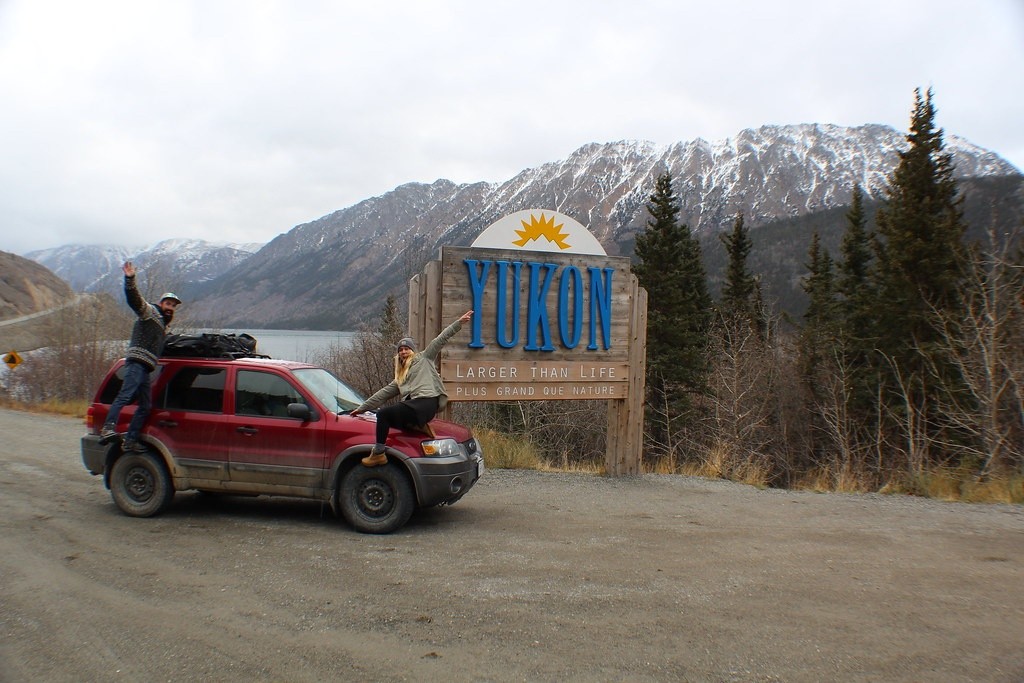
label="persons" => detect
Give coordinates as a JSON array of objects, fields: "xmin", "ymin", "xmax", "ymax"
[
  {"xmin": 349, "ymin": 310, "xmax": 475, "ymax": 467},
  {"xmin": 97, "ymin": 259, "xmax": 182, "ymax": 453}
]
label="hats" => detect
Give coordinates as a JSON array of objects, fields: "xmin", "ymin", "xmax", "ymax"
[
  {"xmin": 160, "ymin": 292, "xmax": 182, "ymax": 305},
  {"xmin": 397, "ymin": 338, "xmax": 415, "ymax": 352}
]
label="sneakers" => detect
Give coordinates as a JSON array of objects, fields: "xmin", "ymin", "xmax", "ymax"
[
  {"xmin": 362, "ymin": 448, "xmax": 387, "ymax": 466},
  {"xmin": 98, "ymin": 422, "xmax": 118, "ymax": 446},
  {"xmin": 121, "ymin": 438, "xmax": 148, "ymax": 452},
  {"xmin": 416, "ymin": 422, "xmax": 436, "ymax": 438}
]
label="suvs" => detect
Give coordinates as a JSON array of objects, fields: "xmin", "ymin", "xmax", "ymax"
[{"xmin": 81, "ymin": 354, "xmax": 486, "ymax": 535}]
